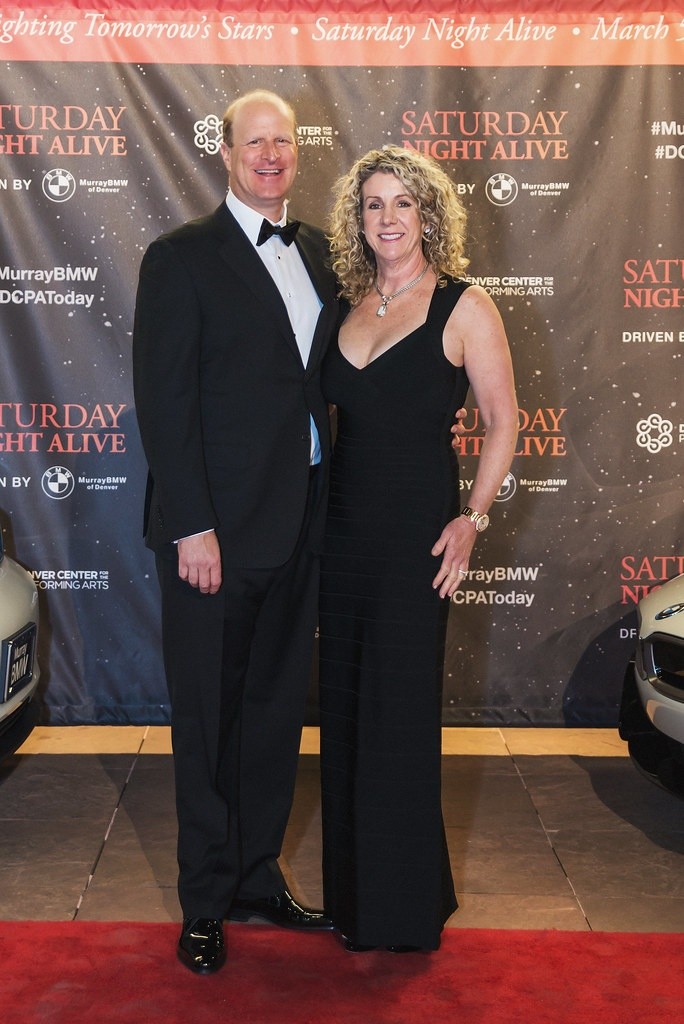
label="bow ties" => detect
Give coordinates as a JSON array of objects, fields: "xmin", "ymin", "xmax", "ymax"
[{"xmin": 256, "ymin": 218, "xmax": 301, "ymax": 247}]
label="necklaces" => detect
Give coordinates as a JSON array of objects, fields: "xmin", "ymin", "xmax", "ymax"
[{"xmin": 370, "ymin": 260, "xmax": 430, "ymax": 318}]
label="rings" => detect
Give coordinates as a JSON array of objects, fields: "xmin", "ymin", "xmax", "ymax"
[{"xmin": 458, "ymin": 569, "xmax": 469, "ymax": 577}]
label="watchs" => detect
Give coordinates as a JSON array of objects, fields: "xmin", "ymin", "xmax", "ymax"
[{"xmin": 460, "ymin": 507, "xmax": 491, "ymax": 533}]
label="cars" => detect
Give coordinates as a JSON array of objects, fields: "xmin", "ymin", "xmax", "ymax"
[
  {"xmin": 0, "ymin": 524, "xmax": 42, "ymax": 768},
  {"xmin": 614, "ymin": 572, "xmax": 684, "ymax": 797}
]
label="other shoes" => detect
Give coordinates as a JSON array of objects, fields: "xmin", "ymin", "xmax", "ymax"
[{"xmin": 341, "ymin": 924, "xmax": 440, "ymax": 952}]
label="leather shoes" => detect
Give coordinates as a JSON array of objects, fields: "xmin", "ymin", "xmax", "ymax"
[
  {"xmin": 225, "ymin": 887, "xmax": 334, "ymax": 930},
  {"xmin": 175, "ymin": 910, "xmax": 226, "ymax": 975}
]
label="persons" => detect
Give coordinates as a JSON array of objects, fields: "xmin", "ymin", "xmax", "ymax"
[
  {"xmin": 135, "ymin": 87, "xmax": 350, "ymax": 972},
  {"xmin": 313, "ymin": 144, "xmax": 519, "ymax": 950}
]
